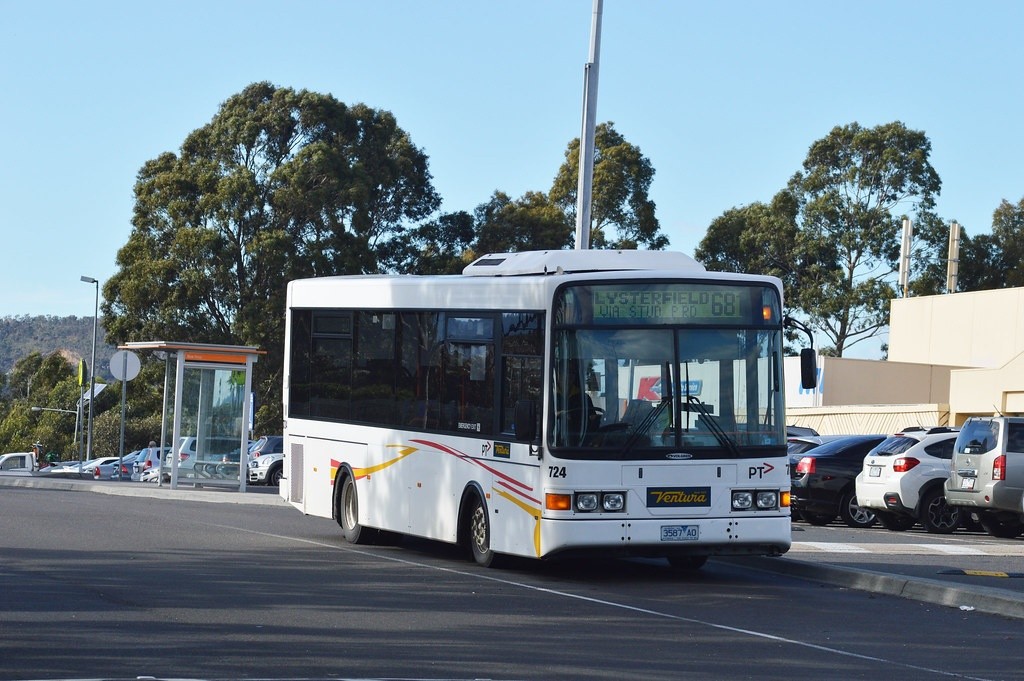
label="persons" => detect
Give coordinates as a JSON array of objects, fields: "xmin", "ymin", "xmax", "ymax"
[
  {"xmin": 148, "ymin": 441, "xmax": 156, "ymax": 448},
  {"xmin": 556, "ymin": 360, "xmax": 600, "ymax": 433}
]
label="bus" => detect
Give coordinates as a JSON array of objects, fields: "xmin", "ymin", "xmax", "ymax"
[{"xmin": 275, "ymin": 244, "xmax": 818, "ymax": 572}]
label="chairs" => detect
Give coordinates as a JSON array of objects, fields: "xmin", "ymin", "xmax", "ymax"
[
  {"xmin": 629, "ymin": 401, "xmax": 652, "ymax": 428},
  {"xmin": 621, "ymin": 399, "xmax": 643, "ymax": 425}
]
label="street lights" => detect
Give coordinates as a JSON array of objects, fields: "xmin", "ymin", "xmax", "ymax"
[
  {"xmin": 31, "ymin": 406, "xmax": 80, "ymax": 460},
  {"xmin": 80, "ymin": 274, "xmax": 99, "ymax": 461}
]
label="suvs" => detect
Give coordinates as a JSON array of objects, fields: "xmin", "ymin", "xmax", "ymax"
[
  {"xmin": 853, "ymin": 425, "xmax": 983, "ymax": 534},
  {"xmin": 942, "ymin": 415, "xmax": 1024, "ymax": 540}
]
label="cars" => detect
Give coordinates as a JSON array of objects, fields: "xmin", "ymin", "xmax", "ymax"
[
  {"xmin": 0, "ymin": 432, "xmax": 284, "ymax": 489},
  {"xmin": 786, "ymin": 433, "xmax": 894, "ymax": 528}
]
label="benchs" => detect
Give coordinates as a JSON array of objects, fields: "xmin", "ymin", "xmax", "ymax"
[{"xmin": 193, "ymin": 461, "xmax": 239, "ymax": 479}]
